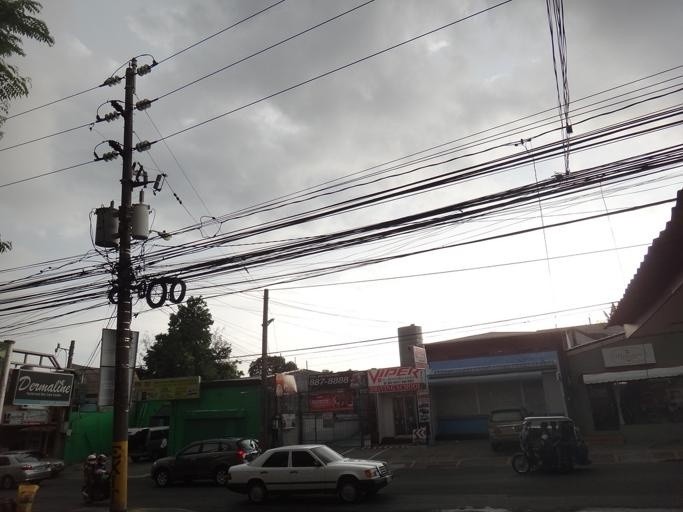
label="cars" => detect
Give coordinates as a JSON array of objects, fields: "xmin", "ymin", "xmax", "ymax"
[
  {"xmin": 226, "ymin": 444, "xmax": 395, "ymax": 506},
  {"xmin": 0, "ymin": 453, "xmax": 53, "ymax": 490},
  {"xmin": 0, "ymin": 449, "xmax": 66, "ymax": 473}
]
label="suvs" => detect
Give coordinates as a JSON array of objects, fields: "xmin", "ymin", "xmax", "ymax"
[{"xmin": 149, "ymin": 437, "xmax": 263, "ymax": 487}]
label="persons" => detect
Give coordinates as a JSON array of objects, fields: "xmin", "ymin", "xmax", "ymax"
[
  {"xmin": 541, "ymin": 422, "xmax": 562, "ymax": 468},
  {"xmin": 161, "ymin": 432, "xmax": 169, "ymax": 457},
  {"xmin": 95, "ymin": 454, "xmax": 107, "ymax": 477},
  {"xmin": 83, "ymin": 454, "xmax": 96, "ymax": 497}
]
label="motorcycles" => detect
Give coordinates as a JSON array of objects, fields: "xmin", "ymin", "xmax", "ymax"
[
  {"xmin": 80, "ymin": 469, "xmax": 111, "ymax": 502},
  {"xmin": 511, "ymin": 415, "xmax": 592, "ymax": 474}
]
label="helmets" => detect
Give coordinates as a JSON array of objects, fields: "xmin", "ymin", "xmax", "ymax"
[
  {"xmin": 87, "ymin": 455, "xmax": 97, "ymax": 464},
  {"xmin": 97, "ymin": 455, "xmax": 106, "ymax": 465}
]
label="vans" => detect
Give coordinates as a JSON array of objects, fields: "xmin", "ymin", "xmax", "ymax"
[
  {"xmin": 486, "ymin": 406, "xmax": 535, "ymax": 451},
  {"xmin": 127, "ymin": 425, "xmax": 170, "ymax": 463}
]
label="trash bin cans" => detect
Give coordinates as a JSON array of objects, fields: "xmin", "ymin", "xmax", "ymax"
[{"xmin": 0, "ymin": 498, "xmax": 16, "ymax": 512}]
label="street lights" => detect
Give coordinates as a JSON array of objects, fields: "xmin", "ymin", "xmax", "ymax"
[{"xmin": 110, "ymin": 228, "xmax": 173, "ymax": 512}]
